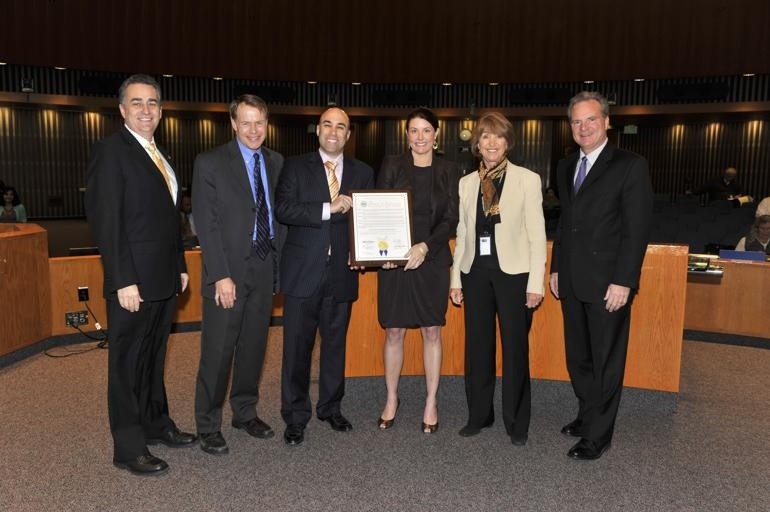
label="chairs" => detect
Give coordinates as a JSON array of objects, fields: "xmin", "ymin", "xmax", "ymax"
[
  {"xmin": 695, "ymin": 206, "xmax": 717, "ymax": 228},
  {"xmin": 698, "ymin": 222, "xmax": 729, "ymax": 242},
  {"xmin": 714, "ymin": 212, "xmax": 734, "ymax": 234},
  {"xmin": 678, "ymin": 215, "xmax": 703, "ymax": 234},
  {"xmin": 661, "ymin": 223, "xmax": 688, "ymax": 247},
  {"xmin": 662, "ymin": 207, "xmax": 685, "ymax": 223},
  {"xmin": 733, "ymin": 208, "xmax": 753, "ymax": 228},
  {"xmin": 723, "ymin": 233, "xmax": 741, "ymax": 248},
  {"xmin": 710, "ymin": 201, "xmax": 734, "ymax": 217},
  {"xmin": 649, "ymin": 214, "xmax": 663, "ymax": 226},
  {"xmin": 677, "ymin": 194, "xmax": 702, "ymax": 214},
  {"xmin": 653, "ymin": 199, "xmax": 669, "ymax": 213},
  {"xmin": 649, "ymin": 230, "xmax": 661, "ymax": 243}
]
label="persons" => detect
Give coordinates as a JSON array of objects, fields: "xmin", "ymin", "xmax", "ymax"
[
  {"xmin": 685, "ymin": 164, "xmax": 742, "ymax": 201},
  {"xmin": 274, "ymin": 105, "xmax": 377, "ymax": 445},
  {"xmin": 549, "ymin": 92, "xmax": 652, "ymax": 464},
  {"xmin": 457, "ymin": 113, "xmax": 548, "ymax": 451},
  {"xmin": 543, "ymin": 187, "xmax": 559, "ymax": 206},
  {"xmin": 734, "ymin": 215, "xmax": 770, "ymax": 256},
  {"xmin": 376, "ymin": 108, "xmax": 459, "ymax": 436},
  {"xmin": 87, "ymin": 72, "xmax": 196, "ymax": 477},
  {"xmin": 181, "ymin": 189, "xmax": 198, "ymax": 245},
  {"xmin": 0, "ymin": 185, "xmax": 28, "ymax": 223},
  {"xmin": 192, "ymin": 93, "xmax": 287, "ymax": 457}
]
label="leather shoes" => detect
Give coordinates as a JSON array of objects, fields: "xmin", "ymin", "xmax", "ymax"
[
  {"xmin": 231, "ymin": 416, "xmax": 274, "ymax": 439},
  {"xmin": 317, "ymin": 412, "xmax": 352, "ymax": 432},
  {"xmin": 511, "ymin": 432, "xmax": 528, "ymax": 446},
  {"xmin": 145, "ymin": 419, "xmax": 197, "ymax": 448},
  {"xmin": 459, "ymin": 424, "xmax": 493, "ymax": 437},
  {"xmin": 113, "ymin": 448, "xmax": 170, "ymax": 478},
  {"xmin": 197, "ymin": 431, "xmax": 229, "ymax": 454},
  {"xmin": 284, "ymin": 423, "xmax": 304, "ymax": 446},
  {"xmin": 561, "ymin": 420, "xmax": 582, "ymax": 435},
  {"xmin": 566, "ymin": 438, "xmax": 608, "ymax": 460}
]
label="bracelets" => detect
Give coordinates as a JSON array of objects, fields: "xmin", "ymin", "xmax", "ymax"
[{"xmin": 415, "ymin": 243, "xmax": 428, "ymax": 256}]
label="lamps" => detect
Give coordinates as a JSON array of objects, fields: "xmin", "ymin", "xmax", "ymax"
[
  {"xmin": 606, "ymin": 92, "xmax": 616, "ymax": 106},
  {"xmin": 457, "ymin": 128, "xmax": 473, "ymax": 152},
  {"xmin": 21, "ymin": 78, "xmax": 35, "ymax": 93},
  {"xmin": 327, "ymin": 94, "xmax": 339, "ymax": 107}
]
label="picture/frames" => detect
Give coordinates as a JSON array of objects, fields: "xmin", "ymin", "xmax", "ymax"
[{"xmin": 347, "ymin": 188, "xmax": 416, "ymax": 268}]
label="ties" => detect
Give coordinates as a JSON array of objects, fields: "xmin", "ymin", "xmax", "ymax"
[
  {"xmin": 325, "ymin": 161, "xmax": 340, "ymax": 204},
  {"xmin": 574, "ymin": 157, "xmax": 587, "ymax": 195},
  {"xmin": 148, "ymin": 143, "xmax": 171, "ymax": 193},
  {"xmin": 253, "ymin": 154, "xmax": 271, "ymax": 260}
]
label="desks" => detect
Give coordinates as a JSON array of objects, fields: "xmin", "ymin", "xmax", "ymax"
[
  {"xmin": 684, "ymin": 253, "xmax": 770, "ymax": 350},
  {"xmin": 0, "ymin": 222, "xmax": 50, "ymax": 368}
]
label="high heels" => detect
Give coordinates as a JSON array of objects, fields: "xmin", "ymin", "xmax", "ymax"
[
  {"xmin": 422, "ymin": 405, "xmax": 438, "ymax": 433},
  {"xmin": 378, "ymin": 398, "xmax": 400, "ymax": 429}
]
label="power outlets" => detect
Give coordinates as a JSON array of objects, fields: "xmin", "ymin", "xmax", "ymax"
[{"xmin": 79, "ymin": 287, "xmax": 90, "ymax": 302}]
